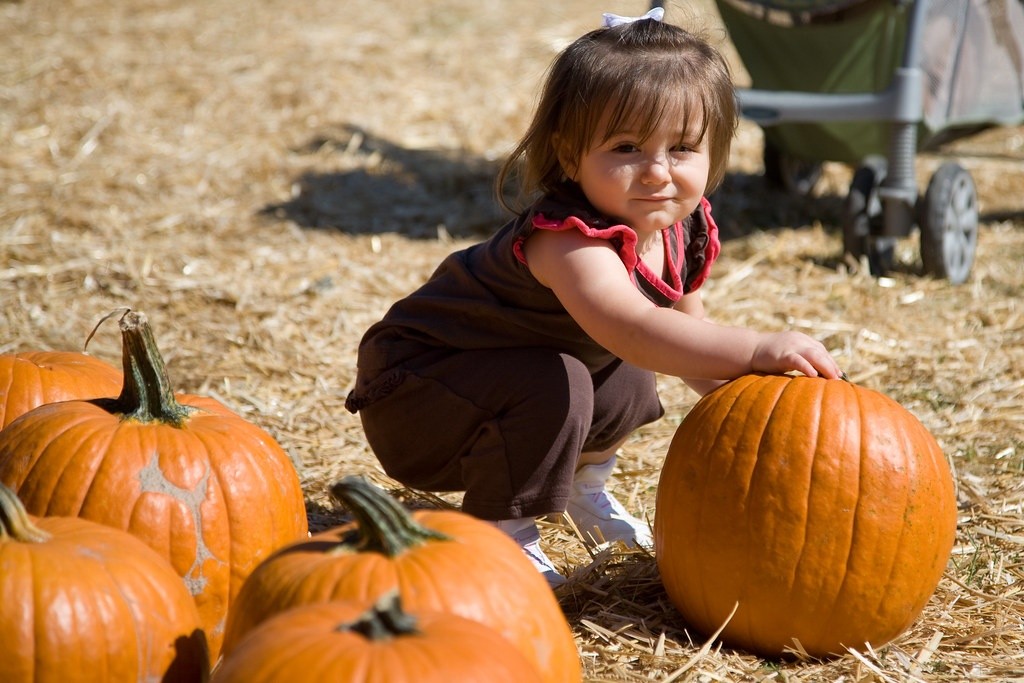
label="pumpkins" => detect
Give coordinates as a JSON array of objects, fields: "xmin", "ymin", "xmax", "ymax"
[
  {"xmin": 654, "ymin": 369, "xmax": 958, "ymax": 660},
  {"xmin": 0, "ymin": 310, "xmax": 584, "ymax": 683}
]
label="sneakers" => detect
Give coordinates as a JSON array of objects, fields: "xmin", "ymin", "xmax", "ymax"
[
  {"xmin": 485, "ymin": 516, "xmax": 568, "ymax": 593},
  {"xmin": 542, "ymin": 456, "xmax": 654, "ymax": 549}
]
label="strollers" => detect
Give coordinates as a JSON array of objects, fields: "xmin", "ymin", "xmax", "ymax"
[{"xmin": 716, "ymin": 0, "xmax": 1024, "ymax": 283}]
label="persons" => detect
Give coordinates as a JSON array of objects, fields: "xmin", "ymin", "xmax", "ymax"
[{"xmin": 344, "ymin": 7, "xmax": 845, "ymax": 591}]
